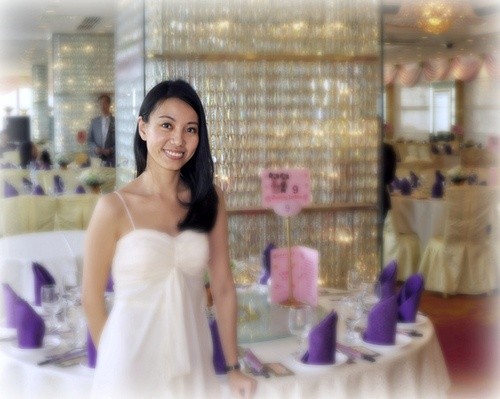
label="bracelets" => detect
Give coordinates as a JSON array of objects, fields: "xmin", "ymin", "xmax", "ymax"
[{"xmin": 225, "ymin": 363, "xmax": 242, "ymax": 372}]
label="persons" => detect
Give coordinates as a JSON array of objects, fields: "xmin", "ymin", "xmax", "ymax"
[
  {"xmin": 383, "ymin": 124, "xmax": 398, "ymax": 239},
  {"xmin": 0, "ymin": 131, "xmax": 10, "ymax": 157},
  {"xmin": 88, "ymin": 93, "xmax": 117, "ymax": 168},
  {"xmin": 18, "ymin": 141, "xmax": 38, "ymax": 168},
  {"xmin": 82, "ymin": 78, "xmax": 258, "ymax": 399}
]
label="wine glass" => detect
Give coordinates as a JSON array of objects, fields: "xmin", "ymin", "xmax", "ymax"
[
  {"xmin": 289, "ymin": 305, "xmax": 313, "ymax": 357},
  {"xmin": 337, "ymin": 293, "xmax": 364, "ymax": 345},
  {"xmin": 39, "ymin": 284, "xmax": 65, "ymax": 328},
  {"xmin": 357, "ymin": 280, "xmax": 382, "ymax": 329},
  {"xmin": 315, "ymin": 263, "xmax": 328, "ymax": 295},
  {"xmin": 248, "ymin": 254, "xmax": 265, "ymax": 288}
]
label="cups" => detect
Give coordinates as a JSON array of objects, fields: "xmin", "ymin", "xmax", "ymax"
[
  {"xmin": 346, "ymin": 269, "xmax": 367, "ymax": 297},
  {"xmin": 62, "ymin": 271, "xmax": 79, "ymax": 296}
]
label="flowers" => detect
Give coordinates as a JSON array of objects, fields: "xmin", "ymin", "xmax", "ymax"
[{"xmin": 78, "ymin": 168, "xmax": 115, "ymax": 184}]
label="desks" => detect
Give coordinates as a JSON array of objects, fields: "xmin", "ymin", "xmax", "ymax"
[
  {"xmin": 0, "ymin": 195, "xmax": 106, "ymax": 237},
  {"xmin": 0, "ymin": 281, "xmax": 451, "ymax": 399},
  {"xmin": 383, "ymin": 187, "xmax": 500, "ymax": 295},
  {"xmin": 0, "ymin": 168, "xmax": 117, "ymax": 193}
]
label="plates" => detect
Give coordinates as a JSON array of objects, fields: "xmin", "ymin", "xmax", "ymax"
[
  {"xmin": 8, "ymin": 337, "xmax": 61, "ymax": 357},
  {"xmin": 360, "ymin": 331, "xmax": 413, "ymax": 350},
  {"xmin": 396, "ymin": 314, "xmax": 428, "ymax": 329},
  {"xmin": 291, "ymin": 348, "xmax": 348, "ymax": 368},
  {"xmin": 0, "ymin": 323, "xmax": 17, "ymax": 338},
  {"xmin": 33, "ymin": 287, "xmax": 81, "ymax": 319}
]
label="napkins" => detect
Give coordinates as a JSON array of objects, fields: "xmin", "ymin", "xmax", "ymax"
[
  {"xmin": 0, "ymin": 174, "xmax": 87, "ymax": 196},
  {"xmin": 391, "ymin": 170, "xmax": 487, "ymax": 198},
  {"xmin": 0, "ymin": 244, "xmax": 423, "ymax": 374}
]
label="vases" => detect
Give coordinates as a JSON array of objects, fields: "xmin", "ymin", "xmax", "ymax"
[{"xmin": 88, "ymin": 183, "xmax": 101, "ymax": 194}]
